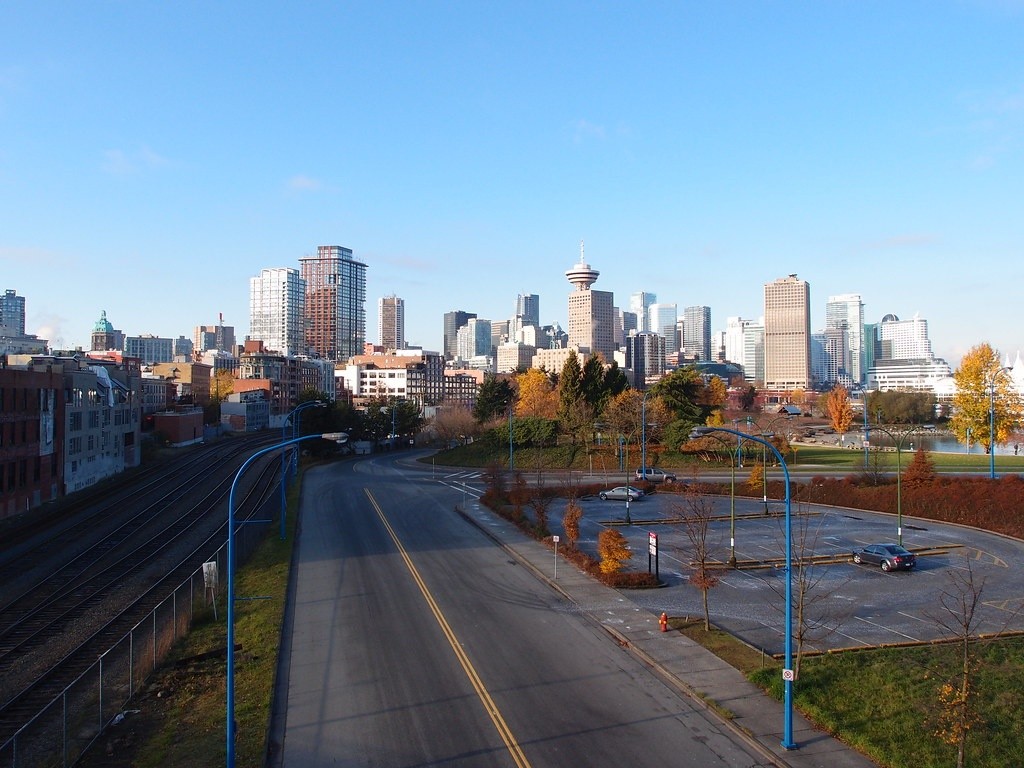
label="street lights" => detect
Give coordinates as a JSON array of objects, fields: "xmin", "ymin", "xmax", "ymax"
[
  {"xmin": 689, "ymin": 426, "xmax": 799, "ymax": 751},
  {"xmin": 859, "ymin": 424, "xmax": 935, "ymax": 545},
  {"xmin": 688, "ymin": 432, "xmax": 775, "ymax": 566},
  {"xmin": 281, "ymin": 404, "xmax": 326, "ymax": 541},
  {"xmin": 625, "ymin": 422, "xmax": 658, "ymax": 523},
  {"xmin": 282, "ymin": 404, "xmax": 319, "ymax": 503},
  {"xmin": 293, "ymin": 399, "xmax": 321, "ymax": 478},
  {"xmin": 392, "ymin": 401, "xmax": 408, "ymax": 439},
  {"xmin": 848, "ymin": 382, "xmax": 868, "ymax": 469},
  {"xmin": 503, "ymin": 401, "xmax": 513, "ymax": 479},
  {"xmin": 226, "ymin": 431, "xmax": 348, "ymax": 766},
  {"xmin": 991, "ymin": 367, "xmax": 1012, "ymax": 479},
  {"xmin": 731, "ymin": 415, "xmax": 793, "ymax": 515}
]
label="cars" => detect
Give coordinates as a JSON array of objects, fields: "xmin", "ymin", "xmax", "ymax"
[
  {"xmin": 449, "ymin": 440, "xmax": 460, "ymax": 449},
  {"xmin": 599, "ymin": 486, "xmax": 645, "ymax": 503},
  {"xmin": 852, "ymin": 543, "xmax": 918, "ymax": 572}
]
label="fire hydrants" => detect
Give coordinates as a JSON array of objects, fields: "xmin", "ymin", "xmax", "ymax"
[{"xmin": 660, "ymin": 612, "xmax": 668, "ymax": 634}]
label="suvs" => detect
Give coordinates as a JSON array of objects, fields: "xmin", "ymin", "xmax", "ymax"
[{"xmin": 635, "ymin": 468, "xmax": 677, "ymax": 484}]
